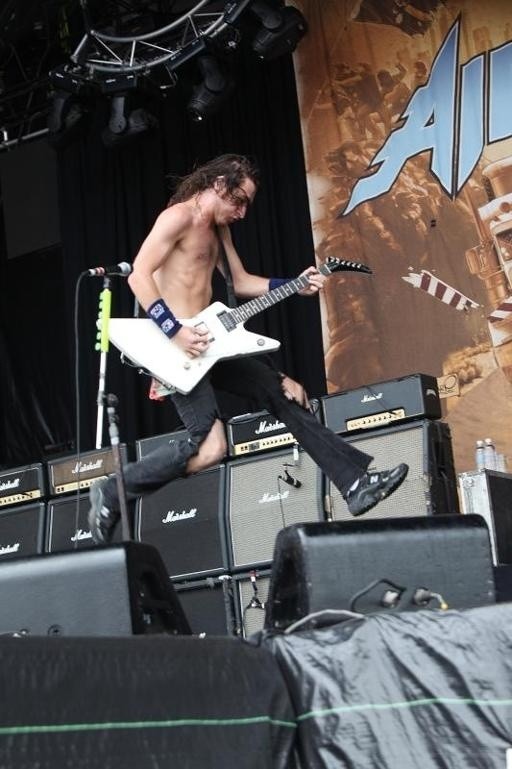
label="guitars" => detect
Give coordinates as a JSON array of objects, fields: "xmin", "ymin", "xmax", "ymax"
[{"xmin": 96, "ymin": 255, "xmax": 373, "ymax": 395}]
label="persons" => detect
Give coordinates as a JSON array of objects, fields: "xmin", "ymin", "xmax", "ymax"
[{"xmin": 87, "ymin": 153, "xmax": 411, "ymax": 548}]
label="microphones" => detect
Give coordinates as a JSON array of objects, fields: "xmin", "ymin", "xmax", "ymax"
[
  {"xmin": 84, "ymin": 260, "xmax": 134, "ymax": 282},
  {"xmin": 278, "ymin": 469, "xmax": 300, "ymax": 490}
]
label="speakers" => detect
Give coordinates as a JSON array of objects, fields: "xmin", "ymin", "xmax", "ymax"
[
  {"xmin": 0, "ymin": 539, "xmax": 194, "ymax": 637},
  {"xmin": 264, "ymin": 514, "xmax": 498, "ymax": 641}
]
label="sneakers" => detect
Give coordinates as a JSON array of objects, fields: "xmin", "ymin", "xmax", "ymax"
[
  {"xmin": 88, "ymin": 479, "xmax": 121, "ymax": 546},
  {"xmin": 343, "ymin": 464, "xmax": 411, "ymax": 517}
]
